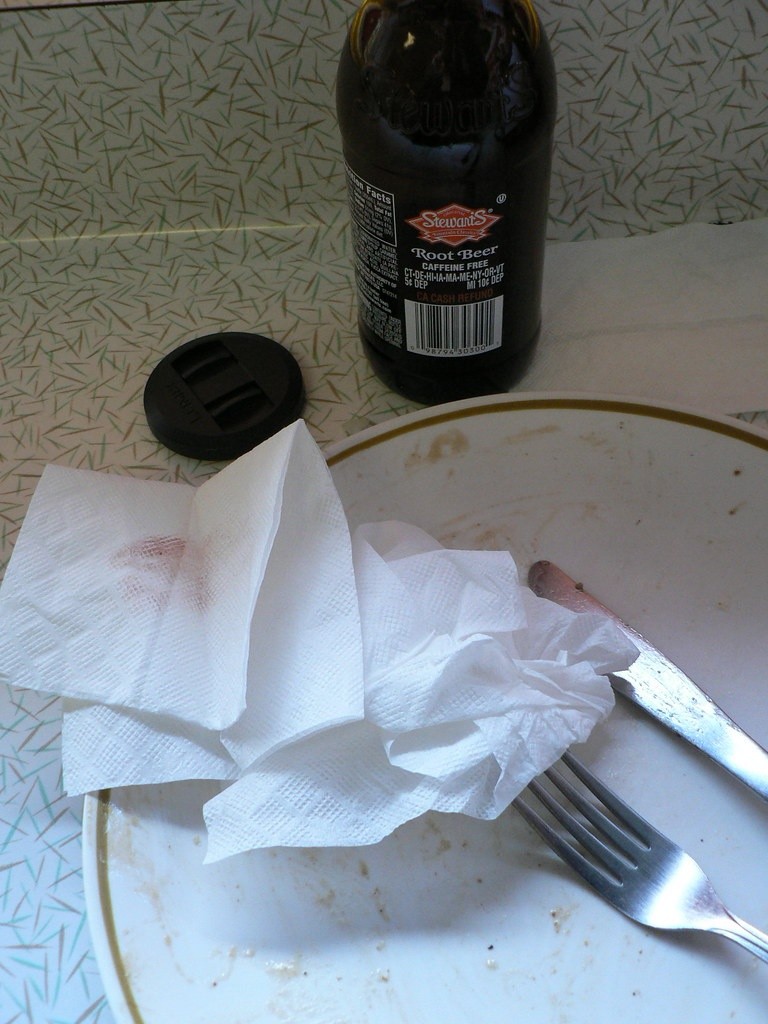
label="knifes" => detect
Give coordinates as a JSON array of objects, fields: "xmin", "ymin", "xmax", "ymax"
[{"xmin": 526, "ymin": 560, "xmax": 768, "ymax": 802}]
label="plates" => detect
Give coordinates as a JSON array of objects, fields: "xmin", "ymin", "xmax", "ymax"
[{"xmin": 82, "ymin": 387, "xmax": 768, "ymax": 1024}]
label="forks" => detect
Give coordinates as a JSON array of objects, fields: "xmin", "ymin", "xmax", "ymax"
[{"xmin": 511, "ymin": 750, "xmax": 768, "ymax": 966}]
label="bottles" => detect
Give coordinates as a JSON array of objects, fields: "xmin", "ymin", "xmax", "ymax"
[{"xmin": 334, "ymin": 0, "xmax": 556, "ymax": 407}]
